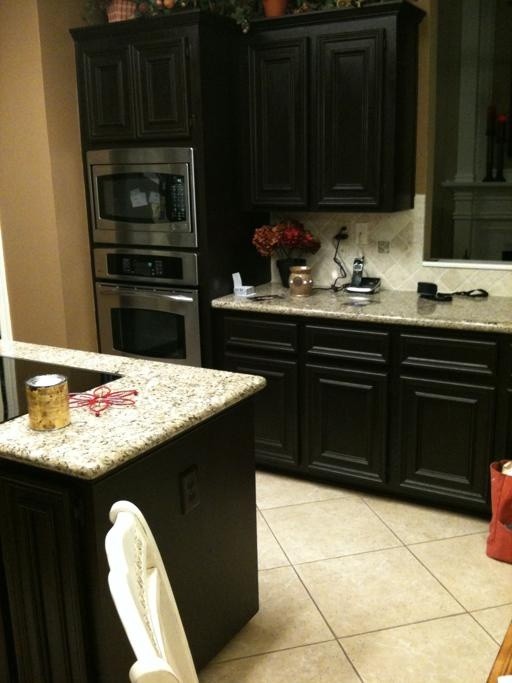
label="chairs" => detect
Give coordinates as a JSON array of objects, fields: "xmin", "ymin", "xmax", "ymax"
[{"xmin": 105, "ymin": 500, "xmax": 201, "ymax": 683}]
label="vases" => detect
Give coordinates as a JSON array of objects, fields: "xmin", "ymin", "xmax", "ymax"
[
  {"xmin": 105, "ymin": 0, "xmax": 137, "ymax": 23},
  {"xmin": 263, "ymin": 0, "xmax": 288, "ymax": 18},
  {"xmin": 275, "ymin": 259, "xmax": 306, "ymax": 288}
]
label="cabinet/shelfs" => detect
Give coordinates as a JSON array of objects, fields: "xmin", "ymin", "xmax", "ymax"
[
  {"xmin": 238, "ymin": 0, "xmax": 426, "ymax": 213},
  {"xmin": 69, "ymin": 9, "xmax": 238, "ymax": 148}
]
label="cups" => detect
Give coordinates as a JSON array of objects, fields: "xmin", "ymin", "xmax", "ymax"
[{"xmin": 287, "ymin": 264, "xmax": 316, "ymax": 300}]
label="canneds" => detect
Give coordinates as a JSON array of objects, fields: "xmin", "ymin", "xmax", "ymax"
[{"xmin": 24, "ymin": 373, "xmax": 71, "ymax": 432}]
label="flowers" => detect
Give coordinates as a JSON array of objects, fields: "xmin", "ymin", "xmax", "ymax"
[
  {"xmin": 256, "ymin": 0, "xmax": 365, "ymax": 15},
  {"xmin": 80, "ymin": 0, "xmax": 250, "ymax": 33},
  {"xmin": 253, "ymin": 222, "xmax": 321, "ymax": 261}
]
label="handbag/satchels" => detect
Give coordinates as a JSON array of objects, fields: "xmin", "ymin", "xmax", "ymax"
[{"xmin": 486, "ymin": 458, "xmax": 512, "ymax": 565}]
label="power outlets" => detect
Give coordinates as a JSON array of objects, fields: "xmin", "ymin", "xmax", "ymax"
[{"xmin": 338, "ymin": 221, "xmax": 352, "ymax": 245}]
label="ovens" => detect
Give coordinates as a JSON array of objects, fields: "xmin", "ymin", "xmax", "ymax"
[{"xmin": 83, "ymin": 147, "xmax": 202, "ymax": 368}]
